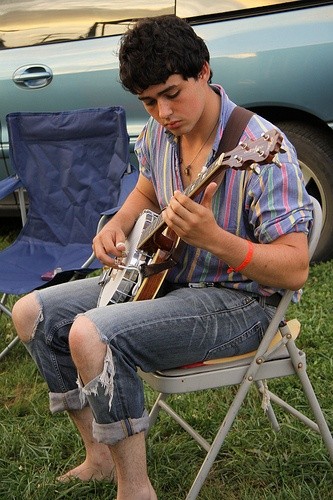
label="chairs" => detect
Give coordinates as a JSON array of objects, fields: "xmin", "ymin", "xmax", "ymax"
[
  {"xmin": 0, "ymin": 106, "xmax": 140, "ymax": 360},
  {"xmin": 144, "ymin": 194, "xmax": 333, "ymax": 500}
]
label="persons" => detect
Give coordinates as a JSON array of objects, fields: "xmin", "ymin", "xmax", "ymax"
[{"xmin": 11, "ymin": 15, "xmax": 314, "ymax": 500}]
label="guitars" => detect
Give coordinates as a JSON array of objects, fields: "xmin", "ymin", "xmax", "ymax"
[{"xmin": 94, "ymin": 128, "xmax": 288, "ymax": 311}]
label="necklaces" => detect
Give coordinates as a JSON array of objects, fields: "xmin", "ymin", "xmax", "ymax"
[{"xmin": 174, "ymin": 123, "xmax": 218, "ymax": 176}]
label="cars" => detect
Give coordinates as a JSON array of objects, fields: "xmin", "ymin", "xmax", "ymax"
[{"xmin": 1, "ymin": 0, "xmax": 333, "ymax": 266}]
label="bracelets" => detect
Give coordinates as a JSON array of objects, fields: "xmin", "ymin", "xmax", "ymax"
[{"xmin": 226, "ymin": 241, "xmax": 254, "ymax": 273}]
